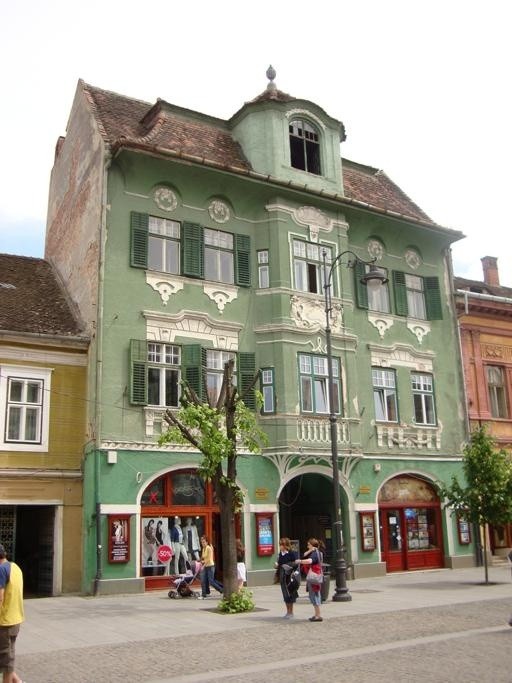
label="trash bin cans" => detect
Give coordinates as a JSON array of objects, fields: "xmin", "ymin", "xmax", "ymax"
[{"xmin": 320, "ymin": 563, "xmax": 331, "ymax": 601}]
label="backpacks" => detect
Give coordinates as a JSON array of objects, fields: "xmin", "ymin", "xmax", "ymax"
[{"xmin": 306, "ymin": 568, "xmax": 323, "ymax": 584}]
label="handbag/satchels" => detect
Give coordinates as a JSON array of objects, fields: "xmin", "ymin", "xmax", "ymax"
[{"xmin": 273, "ymin": 569, "xmax": 280, "ymax": 584}]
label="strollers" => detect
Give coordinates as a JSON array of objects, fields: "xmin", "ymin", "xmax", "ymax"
[{"xmin": 168, "ymin": 559, "xmax": 203, "ymax": 599}]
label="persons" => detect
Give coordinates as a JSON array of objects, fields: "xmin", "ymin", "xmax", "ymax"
[
  {"xmin": 295, "ymin": 538, "xmax": 325, "ymax": 622},
  {"xmin": 200, "ymin": 534, "xmax": 224, "ymax": 595},
  {"xmin": 237, "ymin": 538, "xmax": 247, "ymax": 589},
  {"xmin": 174, "ymin": 569, "xmax": 191, "ymax": 583},
  {"xmin": 197, "ymin": 538, "xmax": 223, "ymax": 599},
  {"xmin": 172, "ymin": 517, "xmax": 188, "ymax": 574},
  {"xmin": 144, "ymin": 519, "xmax": 158, "ymax": 560},
  {"xmin": 272, "ymin": 537, "xmax": 298, "ymax": 618},
  {"xmin": 0, "ymin": 545, "xmax": 24, "ymax": 682},
  {"xmin": 155, "ymin": 520, "xmax": 166, "ymax": 564},
  {"xmin": 183, "ymin": 517, "xmax": 201, "ymax": 560},
  {"xmin": 113, "ymin": 519, "xmax": 125, "ymax": 542}
]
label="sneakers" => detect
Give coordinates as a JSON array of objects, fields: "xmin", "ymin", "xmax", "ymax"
[
  {"xmin": 308, "ymin": 616, "xmax": 323, "ymax": 622},
  {"xmin": 283, "ymin": 613, "xmax": 295, "ymax": 619},
  {"xmin": 197, "ymin": 592, "xmax": 213, "ymax": 600}
]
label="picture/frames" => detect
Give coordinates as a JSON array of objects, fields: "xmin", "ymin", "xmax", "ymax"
[
  {"xmin": 359, "ymin": 511, "xmax": 376, "ymax": 551},
  {"xmin": 108, "ymin": 514, "xmax": 130, "ymax": 563},
  {"xmin": 255, "ymin": 513, "xmax": 274, "ymax": 557},
  {"xmin": 456, "ymin": 509, "xmax": 471, "ymax": 544}
]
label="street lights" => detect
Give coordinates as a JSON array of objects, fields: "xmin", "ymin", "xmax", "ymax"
[{"xmin": 321, "ymin": 248, "xmax": 389, "ymax": 603}]
